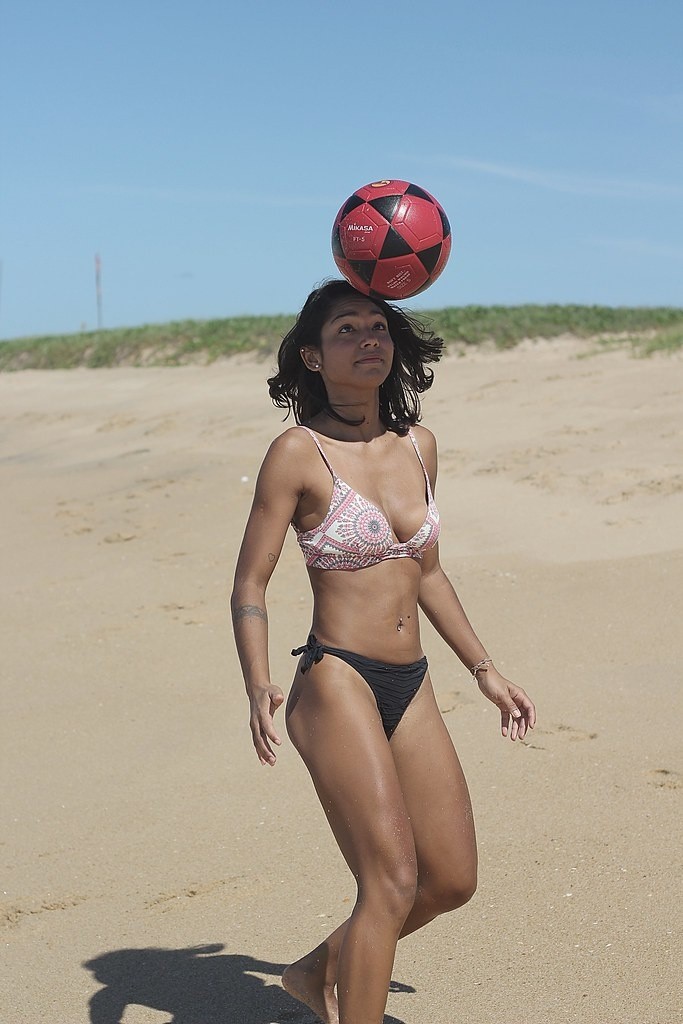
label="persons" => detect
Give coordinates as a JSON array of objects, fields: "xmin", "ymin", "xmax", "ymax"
[{"xmin": 230, "ymin": 280, "xmax": 534, "ymax": 1024}]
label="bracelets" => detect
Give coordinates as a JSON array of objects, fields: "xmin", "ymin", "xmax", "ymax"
[{"xmin": 470, "ymin": 657, "xmax": 492, "ymax": 680}]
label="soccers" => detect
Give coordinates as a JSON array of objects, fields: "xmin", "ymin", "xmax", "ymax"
[{"xmin": 331, "ymin": 177, "xmax": 453, "ymax": 302}]
